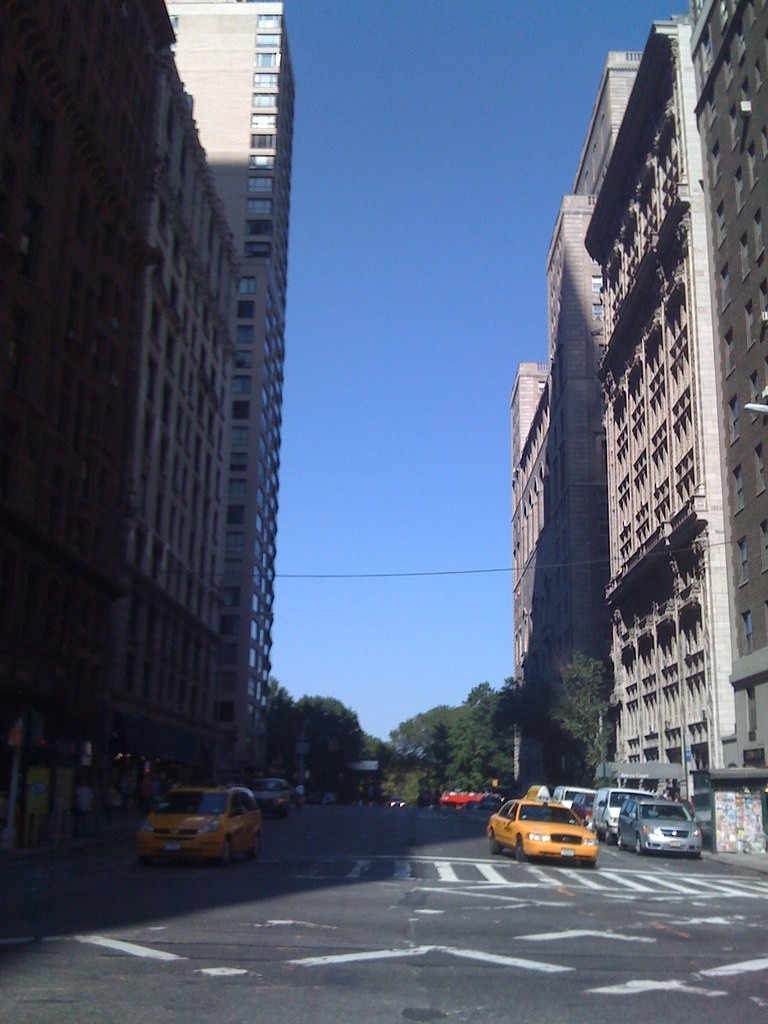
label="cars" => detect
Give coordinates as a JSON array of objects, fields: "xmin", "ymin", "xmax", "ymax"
[
  {"xmin": 244, "ymin": 776, "xmax": 292, "ymax": 819},
  {"xmin": 388, "ymin": 796, "xmax": 406, "ymax": 808},
  {"xmin": 617, "ymin": 798, "xmax": 704, "ymax": 859},
  {"xmin": 135, "ymin": 781, "xmax": 266, "ymax": 868},
  {"xmin": 486, "ymin": 785, "xmax": 600, "ymax": 869}
]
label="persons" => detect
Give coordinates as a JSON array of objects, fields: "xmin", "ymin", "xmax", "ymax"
[
  {"xmin": 121, "ymin": 779, "xmax": 129, "ymax": 815},
  {"xmin": 102, "ymin": 783, "xmax": 113, "ymax": 823},
  {"xmin": 689, "ymin": 795, "xmax": 695, "ymax": 821},
  {"xmin": 655, "ymin": 791, "xmax": 689, "ymax": 818},
  {"xmin": 75, "ymin": 778, "xmax": 96, "ymax": 828},
  {"xmin": 136, "ymin": 774, "xmax": 151, "ymax": 816}
]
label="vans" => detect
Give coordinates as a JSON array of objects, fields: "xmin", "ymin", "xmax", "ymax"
[
  {"xmin": 552, "ymin": 785, "xmax": 599, "ymax": 825},
  {"xmin": 591, "ymin": 787, "xmax": 661, "ymax": 846}
]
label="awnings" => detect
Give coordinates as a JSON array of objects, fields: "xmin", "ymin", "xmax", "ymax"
[{"xmin": 595, "ymin": 761, "xmax": 683, "ymax": 781}]
